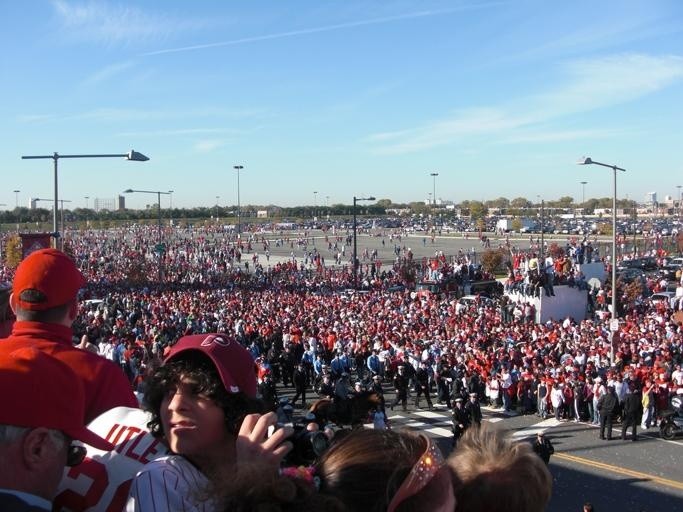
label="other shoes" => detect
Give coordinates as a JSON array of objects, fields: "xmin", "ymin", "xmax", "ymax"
[
  {"xmin": 619, "ymin": 437, "xmax": 626, "ymax": 440},
  {"xmin": 631, "ymin": 438, "xmax": 636, "ymax": 441},
  {"xmin": 391, "ymin": 397, "xmax": 454, "ymax": 410},
  {"xmin": 301, "ymin": 402, "xmax": 306, "ymax": 407},
  {"xmin": 481, "ymin": 400, "xmax": 602, "ymax": 425},
  {"xmin": 600, "ymin": 436, "xmax": 604, "ymax": 440},
  {"xmin": 291, "ymin": 401, "xmax": 296, "ymax": 408}
]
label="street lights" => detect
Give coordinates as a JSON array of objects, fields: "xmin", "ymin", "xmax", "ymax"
[
  {"xmin": 121, "ymin": 188, "xmax": 170, "ymax": 282},
  {"xmin": 13, "ymin": 190, "xmax": 20, "ymax": 207},
  {"xmin": 353, "ymin": 197, "xmax": 376, "ymax": 290},
  {"xmin": 576, "ymin": 156, "xmax": 626, "ymax": 365},
  {"xmin": 430, "ymin": 173, "xmax": 439, "ymax": 205},
  {"xmin": 21, "ymin": 150, "xmax": 150, "ymax": 250},
  {"xmin": 233, "ymin": 165, "xmax": 244, "ymax": 239},
  {"xmin": 578, "ymin": 181, "xmax": 587, "ymax": 203}
]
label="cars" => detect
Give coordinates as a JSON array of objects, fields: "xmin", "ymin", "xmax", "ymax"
[
  {"xmin": 413, "ymin": 280, "xmax": 500, "ymax": 315},
  {"xmin": 617, "ymin": 256, "xmax": 682, "ymax": 304}
]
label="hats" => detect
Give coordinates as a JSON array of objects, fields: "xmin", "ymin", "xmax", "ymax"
[
  {"xmin": 159, "ymin": 332, "xmax": 258, "ymax": 399},
  {"xmin": 470, "ymin": 393, "xmax": 478, "ymax": 398},
  {"xmin": 11, "ymin": 246, "xmax": 89, "ymax": 311},
  {"xmin": 0, "ymin": 347, "xmax": 115, "ymax": 453},
  {"xmin": 455, "ymin": 397, "xmax": 463, "ymax": 403},
  {"xmin": 259, "ymin": 283, "xmax": 682, "ymax": 388}
]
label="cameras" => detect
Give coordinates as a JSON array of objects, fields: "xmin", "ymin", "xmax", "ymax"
[{"xmin": 266, "ymin": 418, "xmax": 329, "ymax": 469}]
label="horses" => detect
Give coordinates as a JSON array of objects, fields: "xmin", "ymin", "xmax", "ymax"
[{"xmin": 309, "ymin": 385, "xmax": 386, "ymax": 431}]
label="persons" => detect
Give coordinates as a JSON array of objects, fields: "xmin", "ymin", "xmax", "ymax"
[{"xmin": 2, "ymin": 214, "xmax": 683, "ymax": 512}]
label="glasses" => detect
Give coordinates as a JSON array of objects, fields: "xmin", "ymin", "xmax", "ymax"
[{"xmin": 65, "ymin": 445, "xmax": 87, "ymax": 468}]
